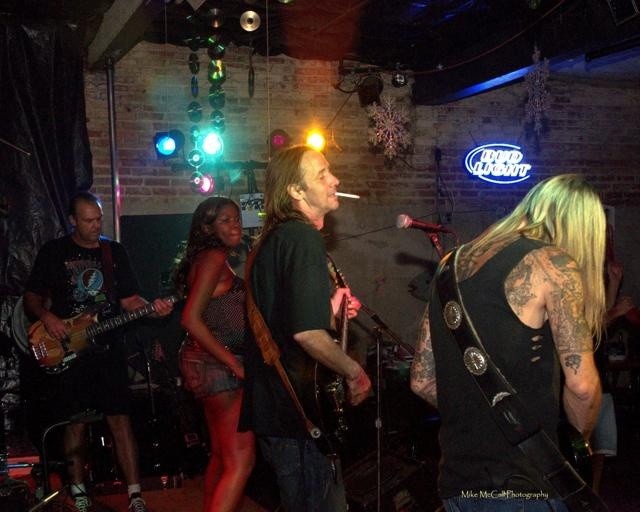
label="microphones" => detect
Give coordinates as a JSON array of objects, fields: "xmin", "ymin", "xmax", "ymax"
[{"xmin": 393, "ymin": 213, "xmax": 452, "ymax": 233}]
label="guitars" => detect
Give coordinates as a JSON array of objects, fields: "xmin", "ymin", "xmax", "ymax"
[
  {"xmin": 311, "ymin": 248, "xmax": 367, "ymax": 456},
  {"xmin": 28, "ymin": 284, "xmax": 192, "ymax": 376}
]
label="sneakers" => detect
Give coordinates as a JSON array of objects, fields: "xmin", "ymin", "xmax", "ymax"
[
  {"xmin": 74, "ymin": 492, "xmax": 93, "ymax": 512},
  {"xmin": 127, "ymin": 492, "xmax": 147, "ymax": 512}
]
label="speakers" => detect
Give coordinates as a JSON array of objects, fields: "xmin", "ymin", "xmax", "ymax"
[{"xmin": 224, "ymin": 0, "xmax": 268, "ymax": 49}]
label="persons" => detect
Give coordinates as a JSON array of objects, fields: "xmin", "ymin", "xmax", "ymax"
[
  {"xmin": 583, "ymin": 224, "xmax": 634, "ymax": 497},
  {"xmin": 171, "ymin": 196, "xmax": 257, "ymax": 511},
  {"xmin": 410, "ymin": 172, "xmax": 610, "ymax": 512},
  {"xmin": 236, "ymin": 143, "xmax": 372, "ymax": 510},
  {"xmin": 23, "ymin": 190, "xmax": 175, "ymax": 512}
]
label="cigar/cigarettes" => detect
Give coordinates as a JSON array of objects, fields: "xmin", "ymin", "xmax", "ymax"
[{"xmin": 334, "ymin": 192, "xmax": 361, "ymax": 200}]
